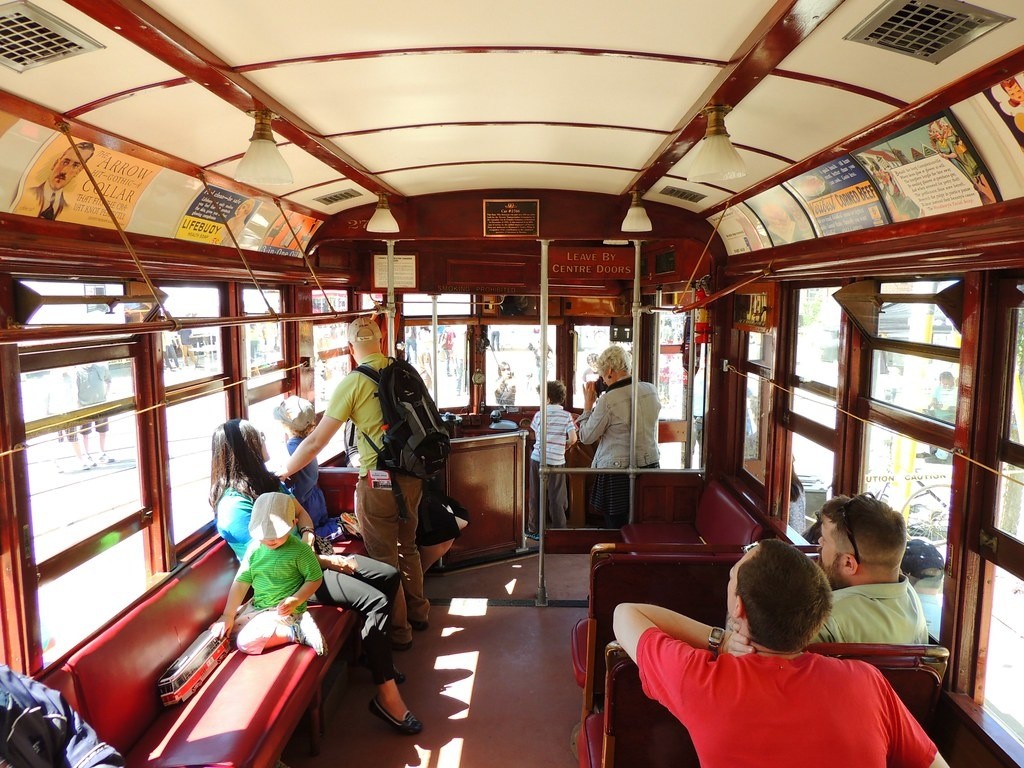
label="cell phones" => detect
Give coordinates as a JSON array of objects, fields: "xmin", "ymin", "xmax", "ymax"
[{"xmin": 344, "ymin": 556, "xmax": 358, "ymax": 569}]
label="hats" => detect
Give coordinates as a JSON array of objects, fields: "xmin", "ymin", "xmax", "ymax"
[
  {"xmin": 348, "ymin": 317, "xmax": 382, "ymax": 342},
  {"xmin": 248, "ymin": 491, "xmax": 295, "ymax": 541},
  {"xmin": 273, "ymin": 395, "xmax": 315, "ymax": 431},
  {"xmin": 902, "ymin": 539, "xmax": 945, "ymax": 581}
]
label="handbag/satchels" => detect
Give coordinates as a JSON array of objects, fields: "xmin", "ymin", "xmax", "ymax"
[
  {"xmin": 314, "ymin": 534, "xmax": 336, "ymax": 556},
  {"xmin": 565, "ymin": 435, "xmax": 601, "ymax": 468}
]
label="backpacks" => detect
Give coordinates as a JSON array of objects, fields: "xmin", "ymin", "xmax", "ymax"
[{"xmin": 350, "ymin": 356, "xmax": 452, "ymax": 481}]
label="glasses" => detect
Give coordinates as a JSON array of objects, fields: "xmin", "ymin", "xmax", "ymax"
[
  {"xmin": 841, "ymin": 492, "xmax": 876, "ymax": 564},
  {"xmin": 501, "ymin": 368, "xmax": 510, "ymax": 371},
  {"xmin": 260, "ymin": 432, "xmax": 266, "ymax": 445}
]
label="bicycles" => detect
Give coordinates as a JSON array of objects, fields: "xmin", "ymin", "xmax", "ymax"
[{"xmin": 898, "ymin": 482, "xmax": 951, "ymax": 546}]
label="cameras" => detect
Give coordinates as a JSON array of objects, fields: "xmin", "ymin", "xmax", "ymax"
[{"xmin": 595, "ymin": 375, "xmax": 609, "ymax": 398}]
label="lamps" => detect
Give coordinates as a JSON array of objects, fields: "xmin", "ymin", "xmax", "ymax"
[
  {"xmin": 234, "ymin": 109, "xmax": 292, "ymax": 185},
  {"xmin": 366, "ymin": 191, "xmax": 399, "ymax": 232},
  {"xmin": 621, "ymin": 190, "xmax": 653, "ymax": 232},
  {"xmin": 686, "ymin": 105, "xmax": 748, "ymax": 182}
]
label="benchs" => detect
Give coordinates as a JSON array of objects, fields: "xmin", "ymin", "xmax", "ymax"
[
  {"xmin": 39, "ymin": 535, "xmax": 370, "ymax": 768},
  {"xmin": 570, "ymin": 478, "xmax": 949, "ymax": 768}
]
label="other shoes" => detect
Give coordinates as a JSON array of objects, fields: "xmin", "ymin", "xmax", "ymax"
[
  {"xmin": 88, "ymin": 455, "xmax": 97, "ymax": 468},
  {"xmin": 917, "ymin": 452, "xmax": 947, "ymax": 463},
  {"xmin": 382, "ymin": 620, "xmax": 429, "ymax": 651},
  {"xmin": 98, "ymin": 453, "xmax": 115, "ymax": 463}
]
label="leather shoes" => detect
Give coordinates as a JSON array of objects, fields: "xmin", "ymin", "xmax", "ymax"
[{"xmin": 368, "ymin": 662, "xmax": 423, "ymax": 735}]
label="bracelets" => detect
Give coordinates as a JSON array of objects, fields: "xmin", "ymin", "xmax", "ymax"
[{"xmin": 300, "ymin": 526, "xmax": 315, "ymax": 537}]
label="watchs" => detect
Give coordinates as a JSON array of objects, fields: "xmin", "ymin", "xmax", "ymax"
[{"xmin": 708, "ymin": 627, "xmax": 724, "ymax": 657}]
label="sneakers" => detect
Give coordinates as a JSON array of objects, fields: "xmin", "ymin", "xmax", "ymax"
[
  {"xmin": 524, "ymin": 526, "xmax": 540, "ymax": 541},
  {"xmin": 299, "ymin": 610, "xmax": 329, "ymax": 657},
  {"xmin": 336, "ymin": 512, "xmax": 364, "ymax": 540}
]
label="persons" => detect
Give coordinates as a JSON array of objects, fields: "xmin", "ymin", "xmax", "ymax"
[
  {"xmin": 613, "ymin": 538, "xmax": 949, "ymax": 768},
  {"xmin": 582, "ymin": 352, "xmax": 600, "ymax": 383},
  {"xmin": 491, "ymin": 331, "xmax": 553, "ymax": 406},
  {"xmin": 39, "ymin": 362, "xmax": 115, "ymax": 473},
  {"xmin": 456, "ymin": 331, "xmax": 468, "ymax": 395},
  {"xmin": 924, "ymin": 371, "xmax": 959, "ymax": 455},
  {"xmin": 405, "ymin": 326, "xmax": 417, "ymax": 365},
  {"xmin": 210, "ymin": 318, "xmax": 469, "ymax": 735},
  {"xmin": 691, "ymin": 358, "xmax": 705, "ymax": 470},
  {"xmin": 522, "ymin": 346, "xmax": 661, "ymax": 541},
  {"xmin": 162, "ymin": 329, "xmax": 205, "ymax": 372},
  {"xmin": 0, "ymin": 664, "xmax": 124, "ymax": 768},
  {"xmin": 440, "ymin": 326, "xmax": 456, "ymax": 377},
  {"xmin": 415, "ymin": 352, "xmax": 433, "ymax": 396},
  {"xmin": 789, "ymin": 456, "xmax": 944, "ymax": 644}
]
